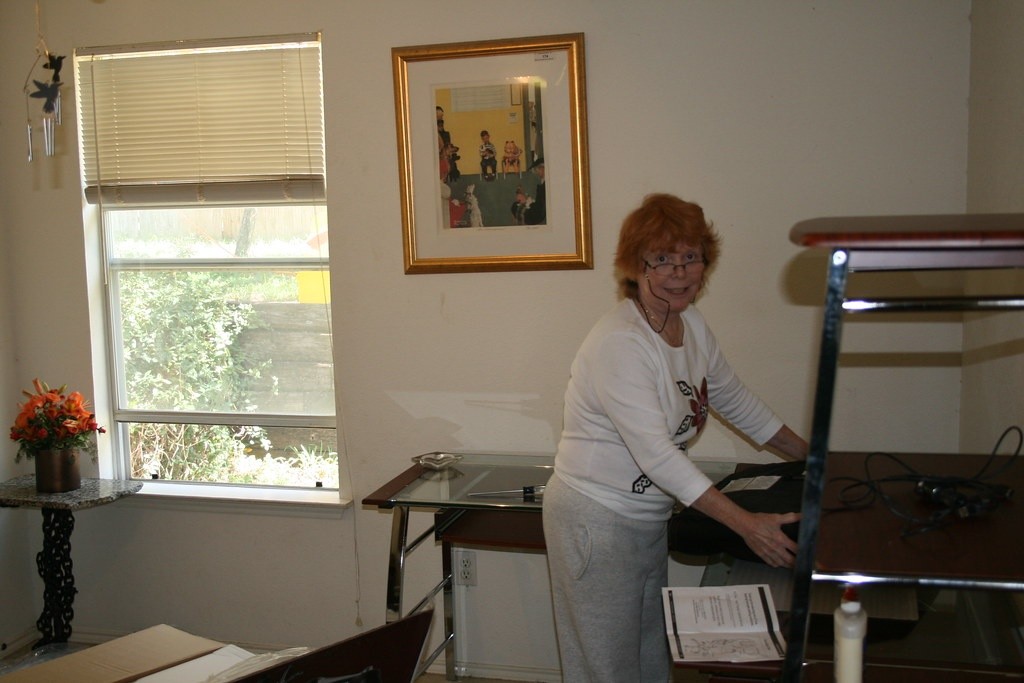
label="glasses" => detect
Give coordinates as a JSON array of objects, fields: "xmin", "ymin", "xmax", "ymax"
[{"xmin": 640, "ymin": 257, "xmax": 708, "ymax": 279}]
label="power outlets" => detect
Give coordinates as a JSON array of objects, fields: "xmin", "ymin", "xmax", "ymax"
[{"xmin": 451, "ymin": 551, "xmax": 477, "ymax": 586}]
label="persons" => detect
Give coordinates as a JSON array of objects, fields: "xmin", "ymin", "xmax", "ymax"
[{"xmin": 542, "ymin": 193, "xmax": 811, "ymax": 682}]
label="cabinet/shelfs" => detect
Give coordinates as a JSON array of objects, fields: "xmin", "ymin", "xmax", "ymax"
[{"xmin": 788, "ymin": 212, "xmax": 1024, "ymax": 683}]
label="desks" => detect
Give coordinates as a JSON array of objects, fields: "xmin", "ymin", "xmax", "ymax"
[
  {"xmin": 361, "ymin": 452, "xmax": 1024, "ymax": 683},
  {"xmin": 0, "ymin": 472, "xmax": 144, "ymax": 650}
]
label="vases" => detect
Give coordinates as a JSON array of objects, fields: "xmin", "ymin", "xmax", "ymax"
[{"xmin": 34, "ymin": 449, "xmax": 81, "ymax": 495}]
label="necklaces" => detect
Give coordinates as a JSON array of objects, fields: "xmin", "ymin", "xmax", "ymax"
[{"xmin": 638, "ymin": 298, "xmax": 666, "ymax": 341}]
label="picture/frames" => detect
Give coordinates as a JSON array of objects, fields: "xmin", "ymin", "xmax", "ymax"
[{"xmin": 391, "ymin": 31, "xmax": 595, "ymax": 275}]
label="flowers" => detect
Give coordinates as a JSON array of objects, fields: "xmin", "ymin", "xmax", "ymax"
[{"xmin": 9, "ymin": 375, "xmax": 105, "ymax": 466}]
